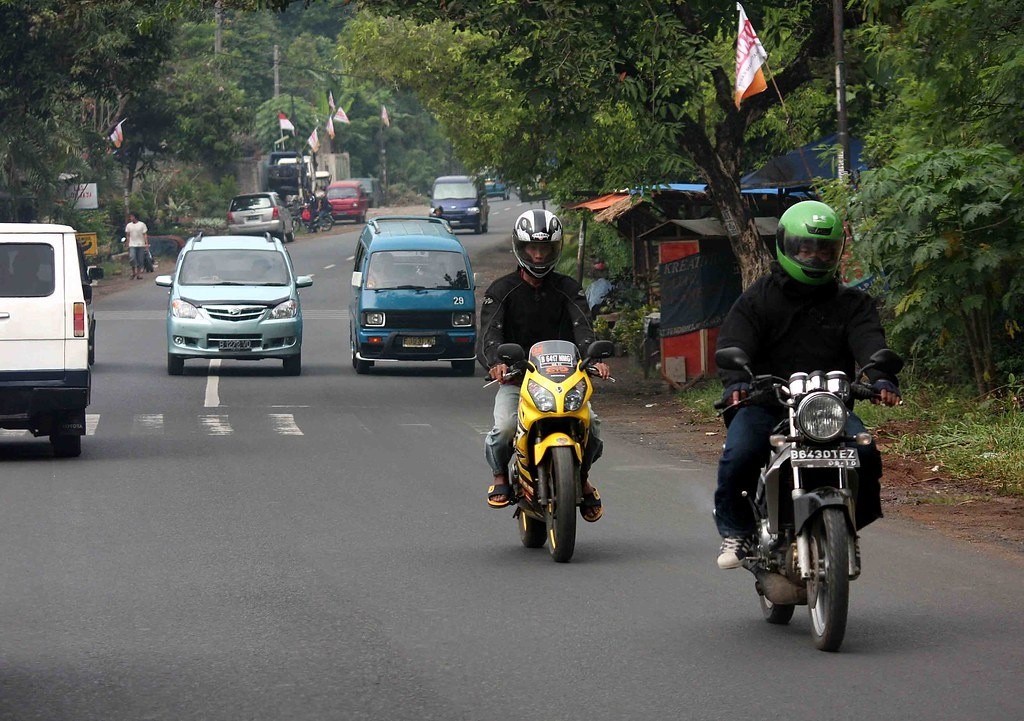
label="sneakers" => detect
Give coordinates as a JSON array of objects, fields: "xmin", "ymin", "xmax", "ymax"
[
  {"xmin": 849, "ymin": 539, "xmax": 861, "ymax": 580},
  {"xmin": 717, "ymin": 535, "xmax": 758, "ymax": 569}
]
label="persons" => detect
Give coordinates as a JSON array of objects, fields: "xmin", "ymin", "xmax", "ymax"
[
  {"xmin": 713, "ymin": 200, "xmax": 901, "ymax": 571},
  {"xmin": 125, "ymin": 211, "xmax": 149, "ymax": 280},
  {"xmin": 476, "ymin": 209, "xmax": 610, "ymax": 522},
  {"xmin": 12, "ymin": 248, "xmax": 49, "ymax": 294},
  {"xmin": 300, "ymin": 186, "xmax": 331, "ymax": 234},
  {"xmin": 429, "ymin": 206, "xmax": 451, "ymax": 225}
]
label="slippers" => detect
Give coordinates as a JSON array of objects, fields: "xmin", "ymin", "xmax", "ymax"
[
  {"xmin": 487, "ymin": 484, "xmax": 513, "ymax": 508},
  {"xmin": 580, "ymin": 488, "xmax": 603, "ymax": 522}
]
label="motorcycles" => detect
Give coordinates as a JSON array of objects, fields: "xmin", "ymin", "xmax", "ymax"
[
  {"xmin": 713, "ymin": 346, "xmax": 904, "ymax": 654},
  {"xmin": 483, "ymin": 340, "xmax": 617, "ymax": 563},
  {"xmin": 290, "ymin": 203, "xmax": 335, "ymax": 234}
]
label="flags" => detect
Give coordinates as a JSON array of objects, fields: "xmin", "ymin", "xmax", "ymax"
[
  {"xmin": 308, "ymin": 129, "xmax": 320, "ymax": 153},
  {"xmin": 734, "ymin": 2, "xmax": 768, "ymax": 111},
  {"xmin": 326, "ymin": 117, "xmax": 335, "ymax": 140}
]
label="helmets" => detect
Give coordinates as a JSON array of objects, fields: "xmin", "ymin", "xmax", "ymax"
[
  {"xmin": 512, "ymin": 209, "xmax": 563, "ymax": 278},
  {"xmin": 776, "ymin": 201, "xmax": 844, "ymax": 284}
]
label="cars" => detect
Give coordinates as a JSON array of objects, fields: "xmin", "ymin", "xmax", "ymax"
[{"xmin": 518, "ymin": 177, "xmax": 553, "ymax": 201}]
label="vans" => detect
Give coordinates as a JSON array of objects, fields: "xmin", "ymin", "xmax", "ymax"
[
  {"xmin": 481, "ymin": 168, "xmax": 510, "ymax": 200},
  {"xmin": 427, "ymin": 176, "xmax": 490, "ymax": 234},
  {"xmin": 228, "ymin": 192, "xmax": 293, "ymax": 242},
  {"xmin": 348, "ymin": 215, "xmax": 477, "ymax": 375},
  {"xmin": 326, "ymin": 180, "xmax": 368, "ymax": 224},
  {"xmin": 157, "ymin": 231, "xmax": 314, "ymax": 376},
  {"xmin": 0, "ymin": 222, "xmax": 104, "ymax": 456}
]
